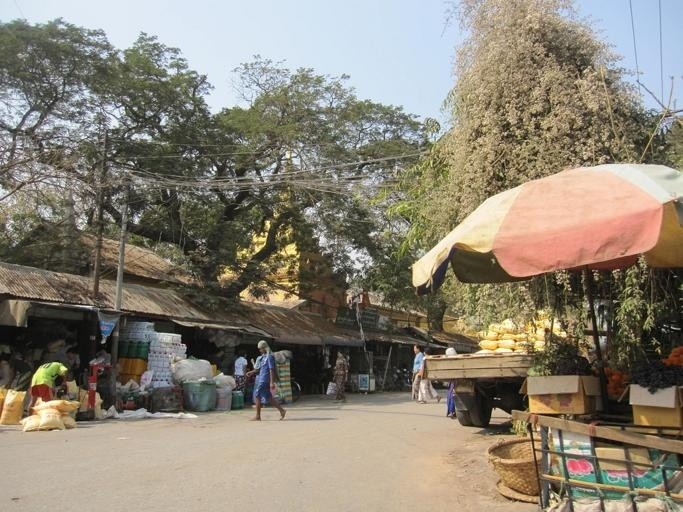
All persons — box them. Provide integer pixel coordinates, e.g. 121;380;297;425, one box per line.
234;349;248;392
332;351;349;402
246;340;286;422
417;347;441;404
66;345;81;370
27;359;69;416
411;344;424;400
445;347;457;419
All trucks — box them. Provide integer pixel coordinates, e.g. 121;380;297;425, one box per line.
424;349;545;429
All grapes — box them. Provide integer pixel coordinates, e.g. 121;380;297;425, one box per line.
636;360;683;394
557;358;586;375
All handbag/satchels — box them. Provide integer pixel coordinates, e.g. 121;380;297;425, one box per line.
326;381;337;396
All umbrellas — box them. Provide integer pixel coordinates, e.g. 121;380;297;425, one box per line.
408;162;683;416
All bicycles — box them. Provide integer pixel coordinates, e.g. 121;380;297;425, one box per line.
290;376;302;403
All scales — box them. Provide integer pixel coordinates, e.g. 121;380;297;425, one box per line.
75;357;105;421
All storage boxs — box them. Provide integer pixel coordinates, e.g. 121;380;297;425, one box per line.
549;425;681;501
615;383;682;431
513;371;604;416
118;319;186;389
